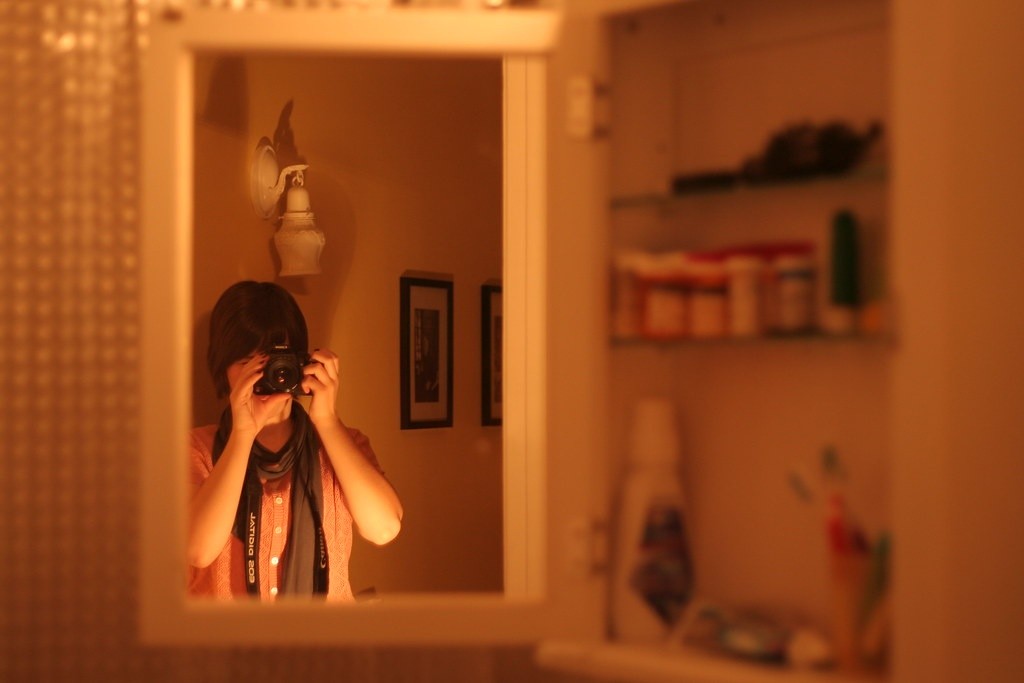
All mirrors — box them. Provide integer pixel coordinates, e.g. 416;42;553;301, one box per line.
139;12;550;651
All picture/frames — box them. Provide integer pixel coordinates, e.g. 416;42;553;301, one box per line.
480;281;503;426
399;275;456;430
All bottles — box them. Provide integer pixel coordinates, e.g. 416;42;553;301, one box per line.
609;391;695;649
609;241;815;336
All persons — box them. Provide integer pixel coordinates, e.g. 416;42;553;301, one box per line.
189;281;404;603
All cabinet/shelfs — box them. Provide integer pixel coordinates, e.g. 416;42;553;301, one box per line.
600;166;887;350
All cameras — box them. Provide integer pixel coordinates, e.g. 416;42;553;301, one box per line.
253;345;324;397
